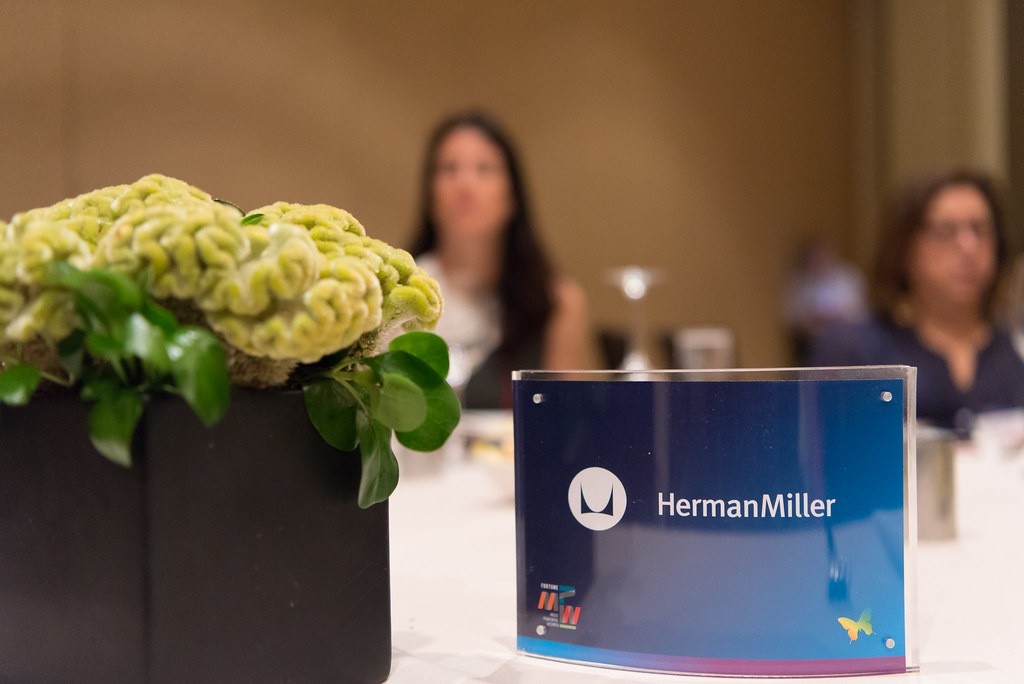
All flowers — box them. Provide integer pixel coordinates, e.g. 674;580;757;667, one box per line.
0;171;462;508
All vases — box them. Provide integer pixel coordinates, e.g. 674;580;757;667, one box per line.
0;388;392;684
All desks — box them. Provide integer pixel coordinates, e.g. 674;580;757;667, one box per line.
388;440;1024;684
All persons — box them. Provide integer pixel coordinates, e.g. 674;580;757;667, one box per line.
801;168;1024;429
377;107;605;410
